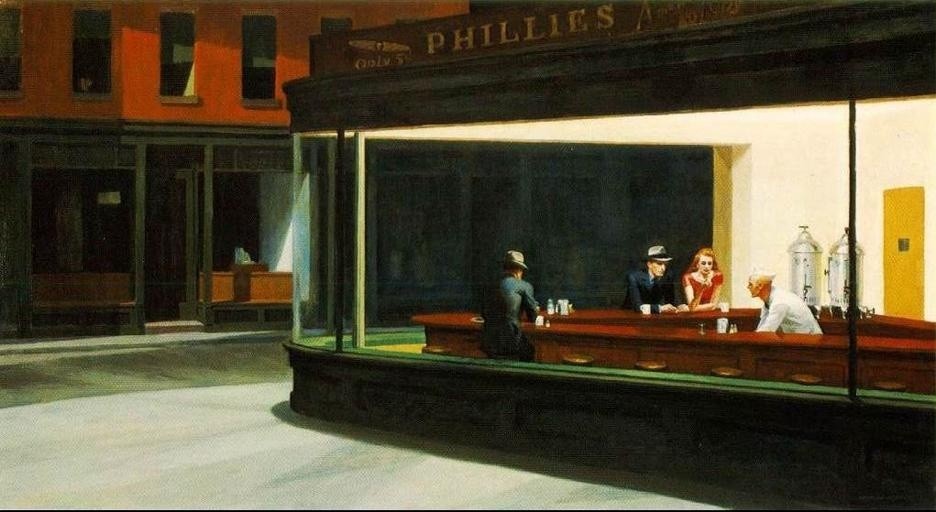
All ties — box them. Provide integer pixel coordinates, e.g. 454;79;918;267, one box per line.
653;278;660;286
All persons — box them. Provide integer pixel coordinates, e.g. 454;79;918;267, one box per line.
745;266;824;335
620;245;690;314
476;250;541;365
679;247;732;314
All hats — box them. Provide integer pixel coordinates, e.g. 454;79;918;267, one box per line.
505;250;528;270
647;245;672;261
749;266;776;280
